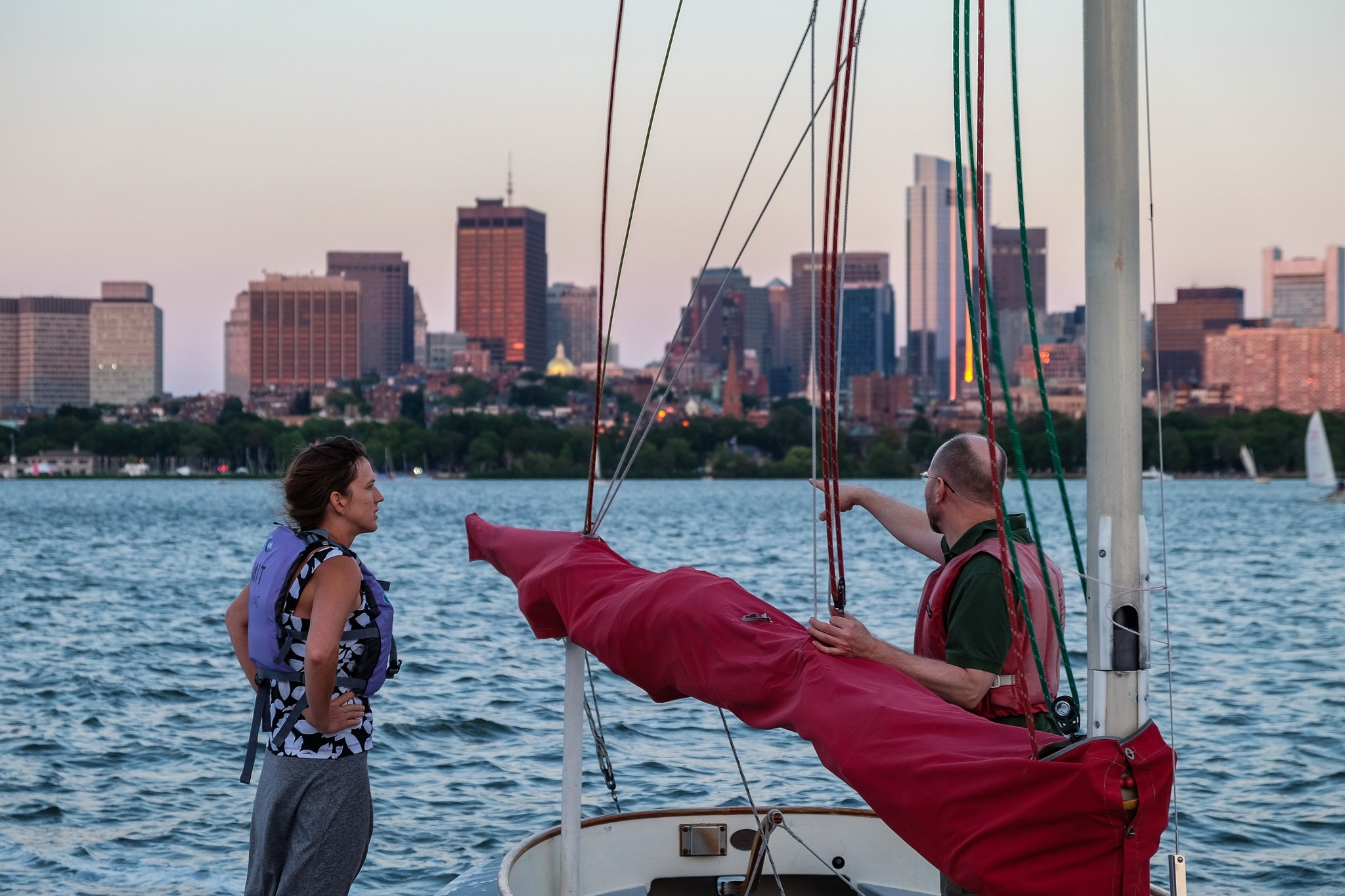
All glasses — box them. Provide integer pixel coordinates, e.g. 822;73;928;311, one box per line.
920;471;955;493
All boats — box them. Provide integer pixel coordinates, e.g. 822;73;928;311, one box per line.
1143;466;1174;481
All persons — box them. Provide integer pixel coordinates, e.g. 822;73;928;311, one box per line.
806;432;1063;896
224;435;404;896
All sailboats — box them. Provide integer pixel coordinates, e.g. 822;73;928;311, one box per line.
431;0;1183;896
1238;444;1269;484
1305;410;1344;505
